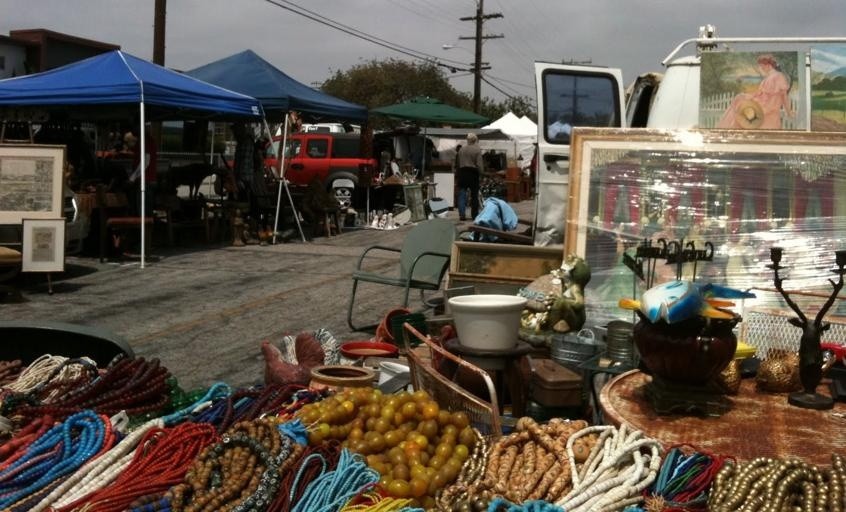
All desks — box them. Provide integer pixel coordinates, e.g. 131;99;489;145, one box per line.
442;335;533;418
69;193;129;236
600;355;846;467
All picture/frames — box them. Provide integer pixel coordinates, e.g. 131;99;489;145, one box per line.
20;216;68;273
0;143;66;226
561;125;846;372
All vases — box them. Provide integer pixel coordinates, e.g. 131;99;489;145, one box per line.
309;308;412;395
448;293;528;349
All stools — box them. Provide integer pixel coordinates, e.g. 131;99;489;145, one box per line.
309;206;347;238
0;246;23;302
105;217;155;259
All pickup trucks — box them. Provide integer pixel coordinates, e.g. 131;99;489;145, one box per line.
215;130;383;199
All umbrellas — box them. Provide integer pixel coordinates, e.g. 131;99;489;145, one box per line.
367;94;490;180
480;106;539;169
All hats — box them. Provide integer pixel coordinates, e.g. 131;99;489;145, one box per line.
734;98;764;130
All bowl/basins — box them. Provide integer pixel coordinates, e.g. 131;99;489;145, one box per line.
339;340;400;361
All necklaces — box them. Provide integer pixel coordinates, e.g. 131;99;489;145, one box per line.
1;347;846;512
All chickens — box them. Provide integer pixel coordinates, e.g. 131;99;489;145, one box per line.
260;331;325;388
279;327;341;366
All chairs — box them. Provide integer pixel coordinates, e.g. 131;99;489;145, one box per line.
0;319;135;367
402;321;528;440
345;217;458;332
155;192;211;244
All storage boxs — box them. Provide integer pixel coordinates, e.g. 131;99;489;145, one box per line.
519;359;583;407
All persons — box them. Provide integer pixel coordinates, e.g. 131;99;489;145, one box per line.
530;146;537;188
230;122;254;199
717;54;800;130
126;114;158;215
453;145;462;185
459;132;485;221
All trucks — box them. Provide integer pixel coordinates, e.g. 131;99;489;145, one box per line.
532;31;845;252
